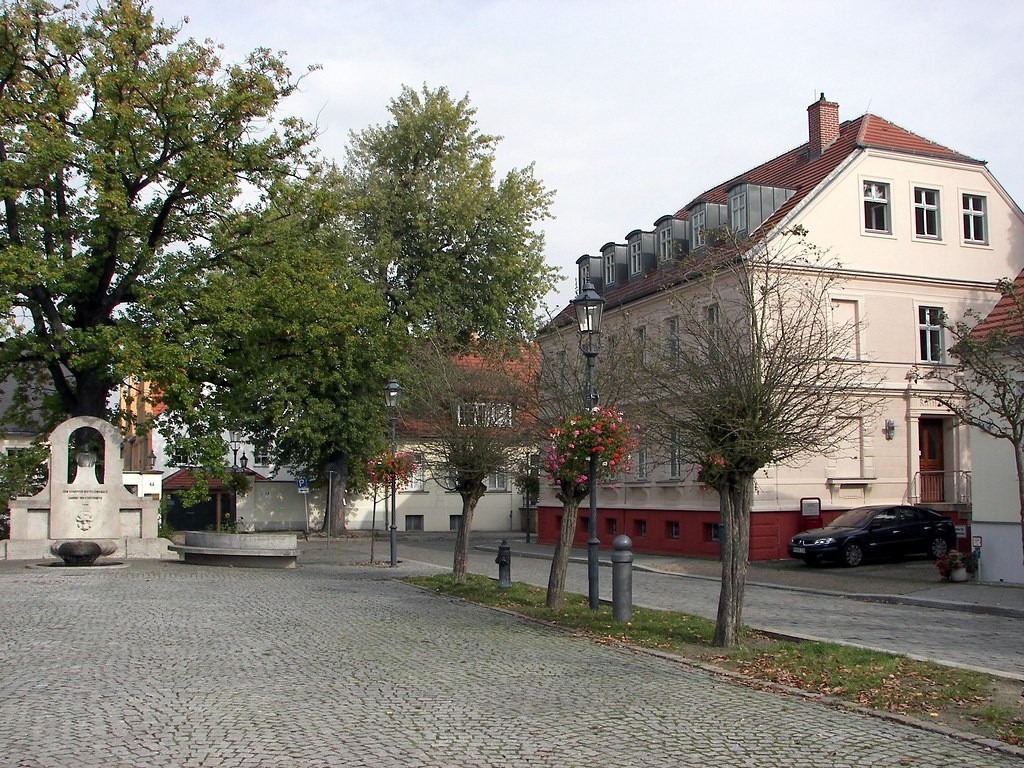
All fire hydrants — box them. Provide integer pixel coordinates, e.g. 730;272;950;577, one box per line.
495;540;511;587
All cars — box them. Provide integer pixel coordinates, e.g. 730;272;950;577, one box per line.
789;503;957;567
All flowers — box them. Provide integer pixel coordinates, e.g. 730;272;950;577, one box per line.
695;449;728;494
935;550;978;576
546;405;641;504
367;451;418;492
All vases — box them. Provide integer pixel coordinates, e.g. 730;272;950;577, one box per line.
950;568;968;581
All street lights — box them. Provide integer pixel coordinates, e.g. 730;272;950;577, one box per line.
569;278;607;612
383;377;403;570
522;446;533;542
230;438;242;533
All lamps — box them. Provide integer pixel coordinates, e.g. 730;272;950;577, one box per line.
886;419;894;440
972;536;982;548
148;450;157;469
